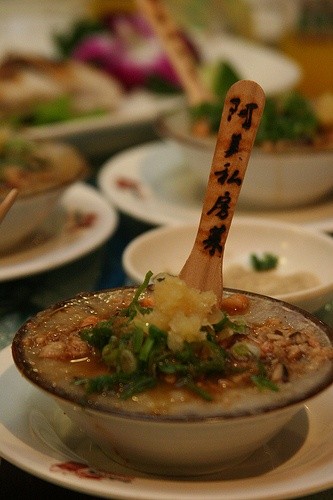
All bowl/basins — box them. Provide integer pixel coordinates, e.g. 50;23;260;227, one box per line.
1;139;89;252
10;284;333;476
122;217;333;315
158;100;333;208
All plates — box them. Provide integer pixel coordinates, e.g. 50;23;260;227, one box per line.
1;344;333;500
96;139;333;233
1;180;119;282
0;13;304;159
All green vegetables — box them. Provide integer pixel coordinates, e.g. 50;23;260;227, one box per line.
187;64;325;141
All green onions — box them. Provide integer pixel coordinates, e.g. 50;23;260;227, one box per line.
71;270;281;402
